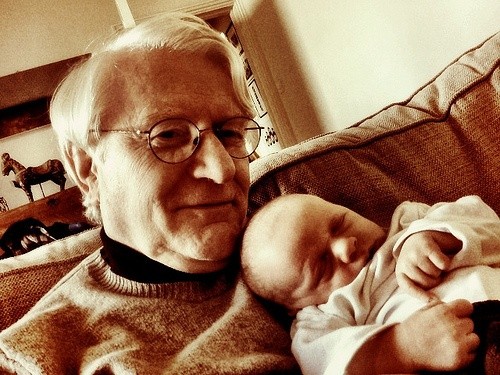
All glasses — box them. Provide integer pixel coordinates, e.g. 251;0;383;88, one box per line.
88;116;264;166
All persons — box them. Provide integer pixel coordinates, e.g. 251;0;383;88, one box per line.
240;193;500;375
0;9;308;375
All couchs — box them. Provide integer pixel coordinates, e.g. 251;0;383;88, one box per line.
1;31;500;374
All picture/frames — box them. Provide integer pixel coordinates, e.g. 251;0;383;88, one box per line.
223;19;245;59
235;51;253;84
245;80;268;119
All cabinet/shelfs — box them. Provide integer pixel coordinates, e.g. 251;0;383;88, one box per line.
1;91;102;261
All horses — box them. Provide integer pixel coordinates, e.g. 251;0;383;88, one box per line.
0;153;66;203
